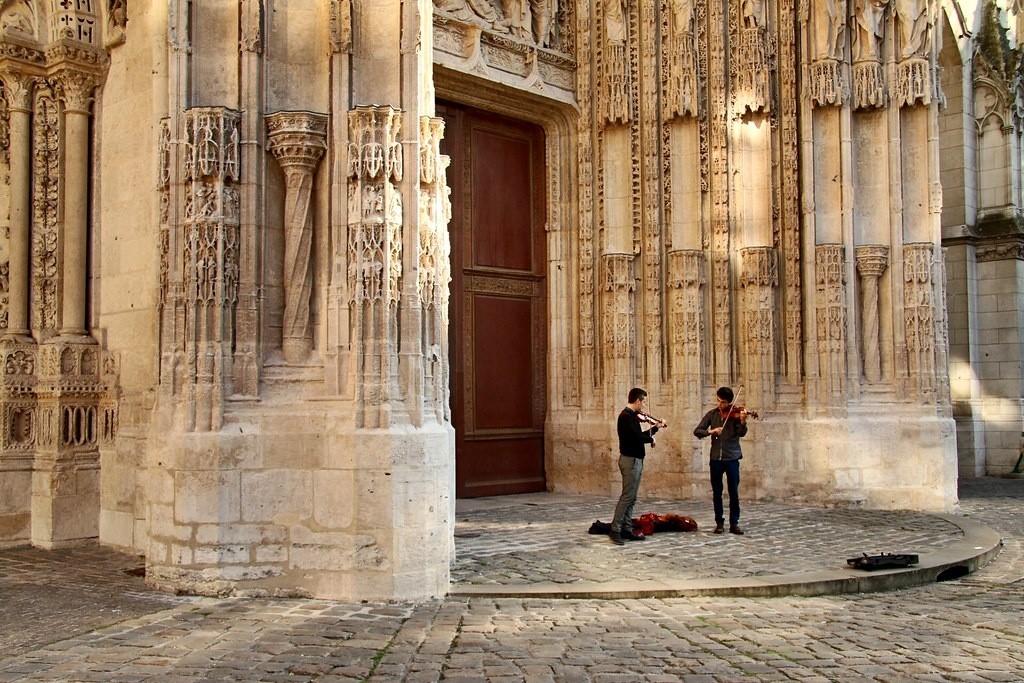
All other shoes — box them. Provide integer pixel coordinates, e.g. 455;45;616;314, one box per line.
714;523;724;533
621;531;640;540
730;523;744;535
609;530;624;545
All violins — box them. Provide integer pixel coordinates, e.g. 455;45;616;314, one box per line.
720;404;759;421
636;410;667;428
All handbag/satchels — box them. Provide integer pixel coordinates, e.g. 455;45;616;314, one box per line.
588;520;610;534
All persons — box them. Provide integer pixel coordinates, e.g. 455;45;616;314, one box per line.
693;386;748;535
609;387;666;546
